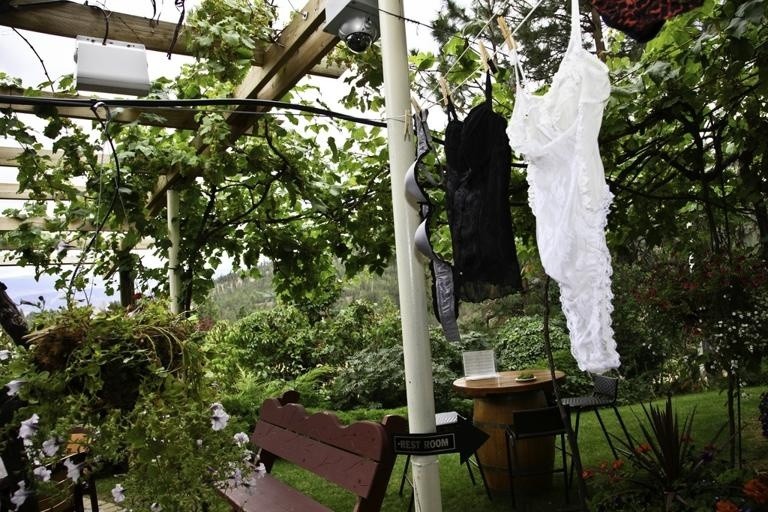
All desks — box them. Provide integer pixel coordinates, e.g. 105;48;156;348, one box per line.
450;366;574;508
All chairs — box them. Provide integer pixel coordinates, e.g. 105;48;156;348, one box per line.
555;371;643;491
0;442;107;512
395;409;481;503
501;404;581;511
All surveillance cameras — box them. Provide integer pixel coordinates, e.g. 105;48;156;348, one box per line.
337;16;378;52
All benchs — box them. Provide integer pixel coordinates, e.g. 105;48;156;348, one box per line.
202;395;406;512
